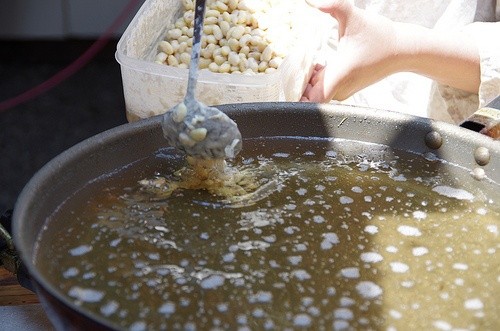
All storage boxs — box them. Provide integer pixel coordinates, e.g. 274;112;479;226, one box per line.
113;0;329;124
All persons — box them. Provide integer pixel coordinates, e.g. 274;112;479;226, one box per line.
299;0;500;126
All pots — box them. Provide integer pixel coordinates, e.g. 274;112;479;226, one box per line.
0;104;500;331
